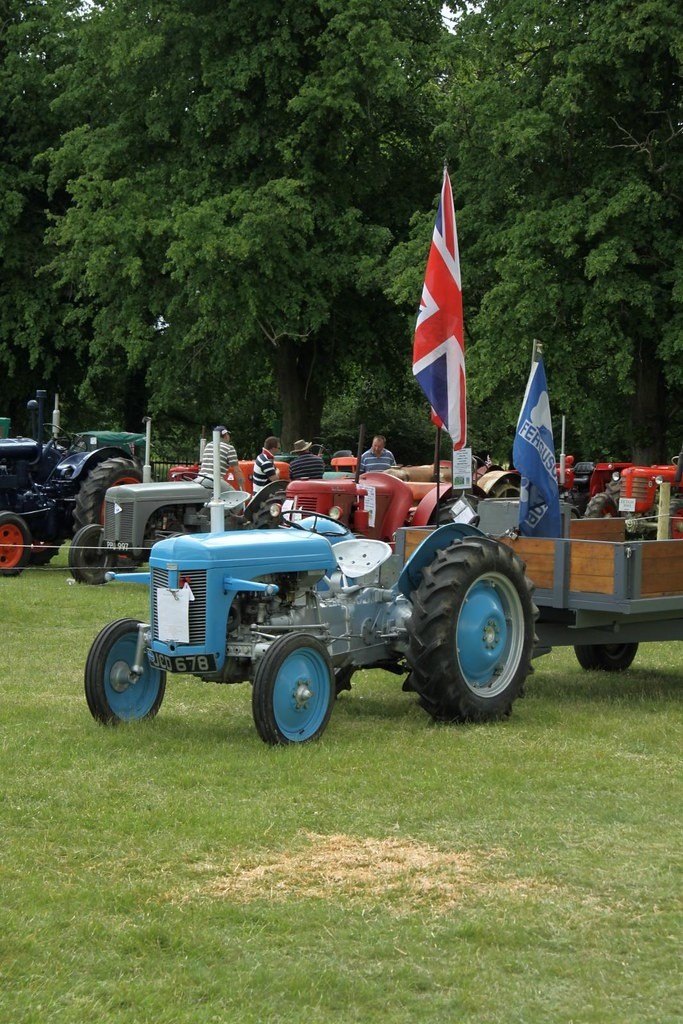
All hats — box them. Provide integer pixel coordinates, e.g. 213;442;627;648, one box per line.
290;440;312;452
214;426;231;436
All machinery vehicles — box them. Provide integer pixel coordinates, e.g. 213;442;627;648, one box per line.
68;417;287;585
581;446;683;539
555;415;634;519
83;431;683;748
0;390;158;577
269;423;487;554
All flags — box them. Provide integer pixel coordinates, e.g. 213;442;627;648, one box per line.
514;358;561;538
412;173;468;452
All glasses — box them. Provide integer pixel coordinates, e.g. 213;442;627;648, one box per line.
276;447;281;450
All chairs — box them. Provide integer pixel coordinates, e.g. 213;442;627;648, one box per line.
332;539;393;594
216;491;252;514
57;452;91;480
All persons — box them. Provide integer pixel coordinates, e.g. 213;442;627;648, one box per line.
253;436;282;496
198;426;246;514
288;439;324;480
359;435;397;473
